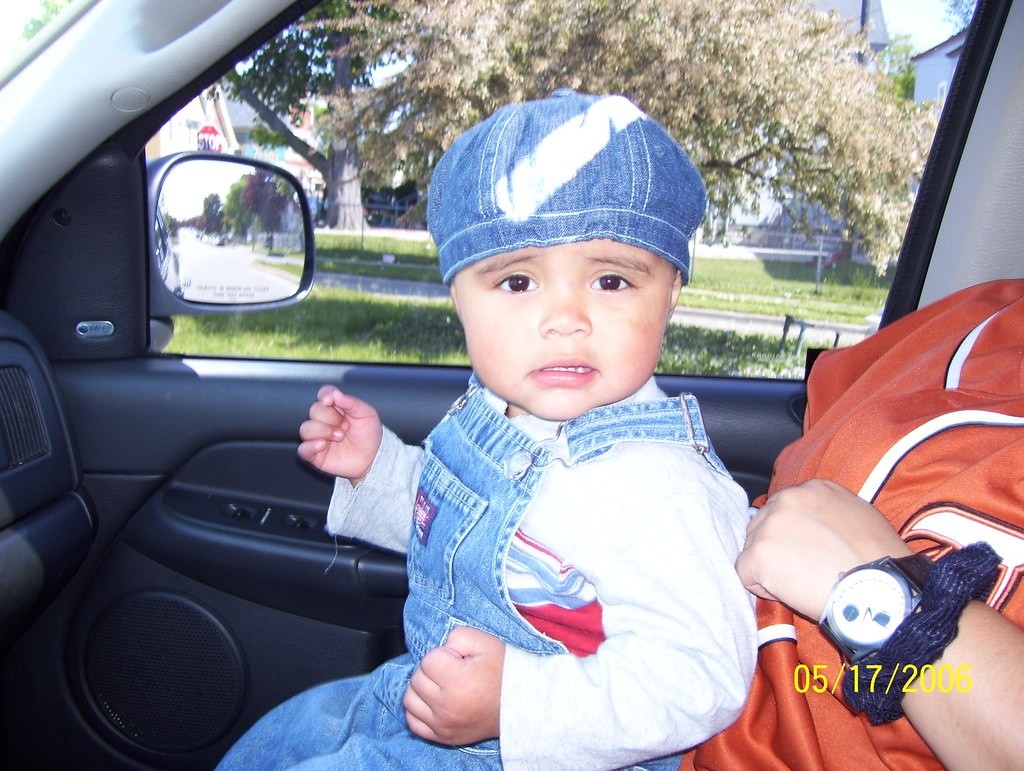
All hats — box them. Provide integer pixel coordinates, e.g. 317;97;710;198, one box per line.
427;89;707;288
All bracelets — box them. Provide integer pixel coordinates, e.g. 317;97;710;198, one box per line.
843;542;997;724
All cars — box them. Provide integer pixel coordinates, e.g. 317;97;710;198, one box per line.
155;207;184;299
214;235;224;246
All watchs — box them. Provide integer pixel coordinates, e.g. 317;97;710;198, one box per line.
818;554;931;663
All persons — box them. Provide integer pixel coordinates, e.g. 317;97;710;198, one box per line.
678;278;1024;771
217;90;759;771
